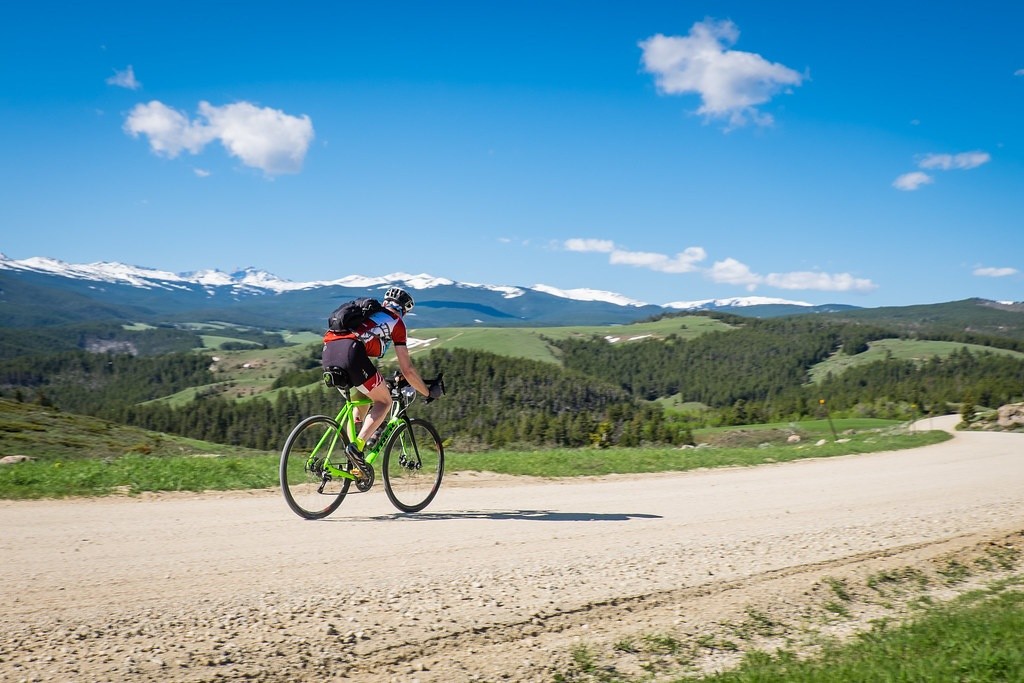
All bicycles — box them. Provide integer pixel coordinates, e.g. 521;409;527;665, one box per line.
278;367;447;520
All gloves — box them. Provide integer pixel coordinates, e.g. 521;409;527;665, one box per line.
428;385;442;399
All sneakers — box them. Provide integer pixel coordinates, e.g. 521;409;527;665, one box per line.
346;444;368;477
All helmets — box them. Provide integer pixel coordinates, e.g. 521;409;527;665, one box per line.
384;287;414;312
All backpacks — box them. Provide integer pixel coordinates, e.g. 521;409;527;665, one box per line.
327;297;397;349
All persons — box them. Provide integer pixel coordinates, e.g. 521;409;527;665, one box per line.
321;286;441;479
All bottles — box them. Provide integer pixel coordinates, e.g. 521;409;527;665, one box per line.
367;421;388;449
